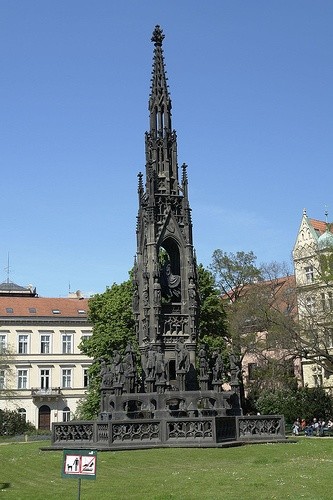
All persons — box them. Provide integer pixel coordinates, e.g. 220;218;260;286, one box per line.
307;418;325;437
99;337;167;386
292;418;300;435
133;255;197;307
326;419;333;431
197;344;241;383
300;418;306;431
175;336;189;370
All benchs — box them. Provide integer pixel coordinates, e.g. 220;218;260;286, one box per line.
292;425;333;436
